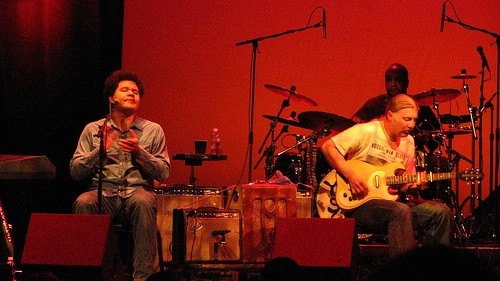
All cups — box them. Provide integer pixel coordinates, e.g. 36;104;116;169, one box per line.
194;140;207;154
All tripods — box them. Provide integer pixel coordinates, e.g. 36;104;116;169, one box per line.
446;64;499;245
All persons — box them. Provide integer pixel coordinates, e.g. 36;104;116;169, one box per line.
70;70;171;281
351;63;442;152
320;94;452;259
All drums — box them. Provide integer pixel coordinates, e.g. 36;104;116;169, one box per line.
272;152;303;180
419;152;452;197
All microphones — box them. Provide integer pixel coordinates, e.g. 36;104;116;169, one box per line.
108;99;119;116
210;230;231;235
440;5;446;31
323;10;326;38
281;110;298;133
477;47;492;71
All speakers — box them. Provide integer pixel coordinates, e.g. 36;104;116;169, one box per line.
155;188;226;263
18;212;120;281
271;218;358;279
183;211;241;262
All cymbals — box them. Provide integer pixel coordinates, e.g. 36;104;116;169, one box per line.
450;75;477;79
430;126;476;138
298;111;357;131
263;84;318;107
261;113;313;130
412;87;462;104
441;114;477;123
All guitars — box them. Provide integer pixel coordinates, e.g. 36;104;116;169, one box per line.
334;160;485;211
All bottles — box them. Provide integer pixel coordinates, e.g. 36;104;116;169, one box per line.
211;128;221;155
